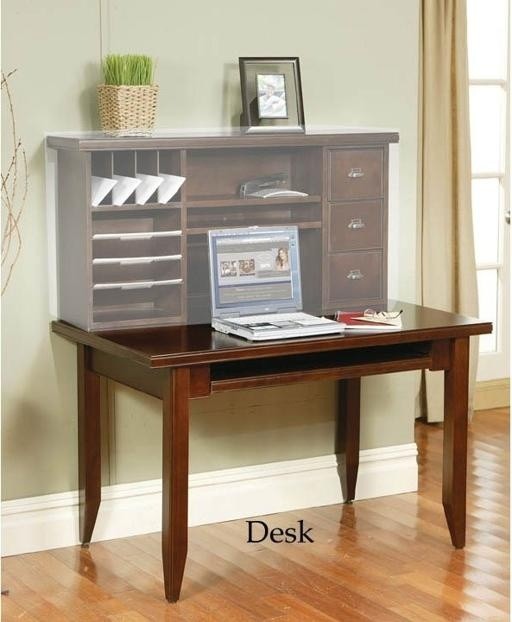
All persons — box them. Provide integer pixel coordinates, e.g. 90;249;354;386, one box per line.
274;247;290;271
259;85;286;112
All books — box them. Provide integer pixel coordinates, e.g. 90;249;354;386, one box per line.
335;310;402;331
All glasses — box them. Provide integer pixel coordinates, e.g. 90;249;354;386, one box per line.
363;307;404;320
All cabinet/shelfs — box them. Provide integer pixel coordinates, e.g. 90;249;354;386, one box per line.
46;129;403;335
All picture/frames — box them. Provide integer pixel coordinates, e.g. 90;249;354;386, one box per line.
237;55;306;135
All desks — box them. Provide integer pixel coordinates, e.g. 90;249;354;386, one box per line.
49;297;495;605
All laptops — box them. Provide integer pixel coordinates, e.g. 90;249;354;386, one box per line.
207;226;347;342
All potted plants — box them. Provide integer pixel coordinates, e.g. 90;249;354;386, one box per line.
95;51;160;138
